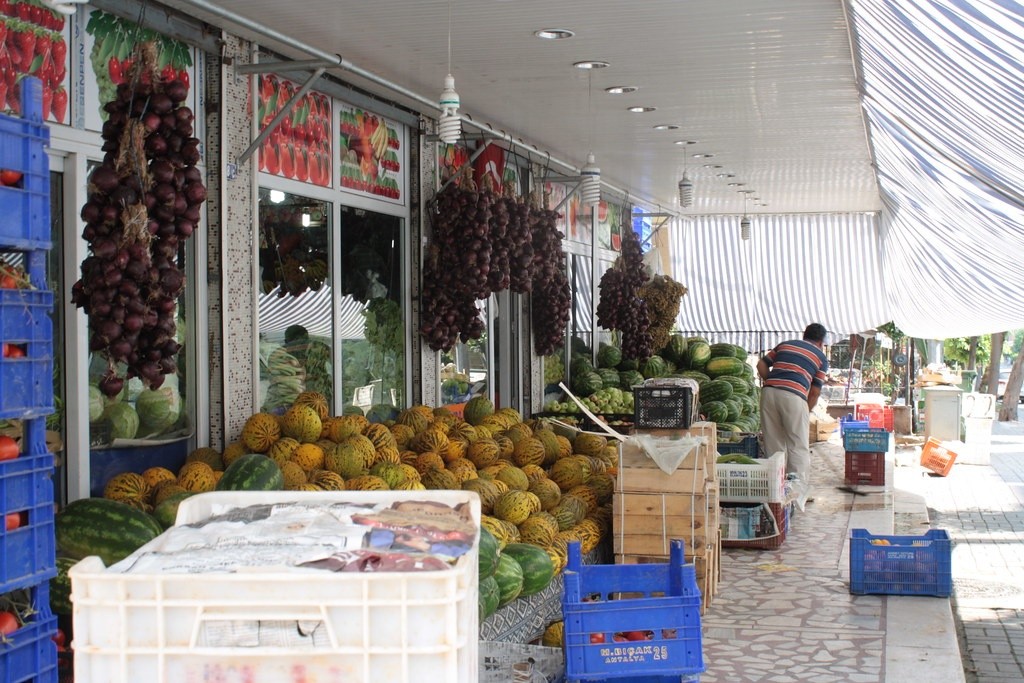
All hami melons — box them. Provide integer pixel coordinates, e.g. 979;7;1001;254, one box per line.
102;392;621;647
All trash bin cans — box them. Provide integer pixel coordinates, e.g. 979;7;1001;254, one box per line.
963;391;995;465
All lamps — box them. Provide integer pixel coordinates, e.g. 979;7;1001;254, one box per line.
740;194;749;242
678;145;693;209
438;0;461;144
581;70;600;206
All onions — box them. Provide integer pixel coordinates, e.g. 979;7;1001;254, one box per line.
71;80;207;399
420;176;571;357
597;231;655;361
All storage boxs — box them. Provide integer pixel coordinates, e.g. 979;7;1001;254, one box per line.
67;491;481;683
0;416;58;594
0;76;54;250
0;251;55;419
0;580;58;683
613;421;722;616
716;452;785;502
845;427;888;452
849;528;951;597
920;436;957;477
560;539;706;680
840;404;894;438
633;385;700;428
719;502;786;551
844;451;885;486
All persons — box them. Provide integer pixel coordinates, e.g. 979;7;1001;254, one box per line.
757;323;827;502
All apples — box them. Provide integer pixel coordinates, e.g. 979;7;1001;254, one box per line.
545;386;635;427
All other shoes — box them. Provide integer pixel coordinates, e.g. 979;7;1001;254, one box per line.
805;495;814;502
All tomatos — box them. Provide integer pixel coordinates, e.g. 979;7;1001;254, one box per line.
0;167;68;656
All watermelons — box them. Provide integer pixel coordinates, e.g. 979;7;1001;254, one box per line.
42;453;554;630
570;334;763;443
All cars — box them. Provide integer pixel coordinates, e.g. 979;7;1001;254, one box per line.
997;369;1024;404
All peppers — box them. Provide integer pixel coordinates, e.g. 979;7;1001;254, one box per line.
338;107;400;199
0;0;189;124
245;73;332;187
